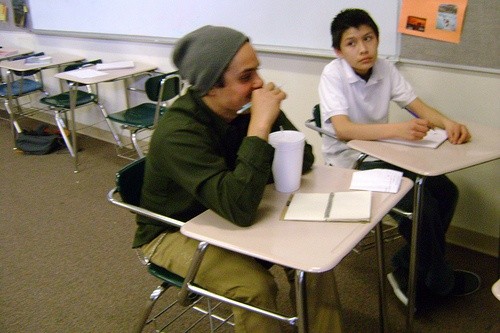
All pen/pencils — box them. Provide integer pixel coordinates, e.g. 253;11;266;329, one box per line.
404;107;435;131
236;83;282;114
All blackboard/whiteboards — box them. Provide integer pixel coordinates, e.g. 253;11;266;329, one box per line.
24;1;401;66
399;1;499;75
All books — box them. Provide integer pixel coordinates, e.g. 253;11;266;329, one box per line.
381;128;449;148
24;57;51;64
280;191;371;222
97;61;134;69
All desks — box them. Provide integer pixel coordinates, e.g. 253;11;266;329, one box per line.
0;45;500;333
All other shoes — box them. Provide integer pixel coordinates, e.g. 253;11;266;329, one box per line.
387;268;481;313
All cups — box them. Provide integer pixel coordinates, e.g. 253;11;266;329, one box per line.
268;131;306;193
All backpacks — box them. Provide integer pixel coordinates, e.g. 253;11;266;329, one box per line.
16;125;70;155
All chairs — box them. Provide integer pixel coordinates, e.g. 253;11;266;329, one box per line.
107;70;184;159
107;157;235;333
39;59;122;159
0;51;52;133
304;104;403;252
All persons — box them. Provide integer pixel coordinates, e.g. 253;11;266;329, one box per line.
132;25;346;333
319;9;482;312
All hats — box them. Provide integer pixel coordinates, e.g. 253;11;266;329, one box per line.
172;25;249;98
